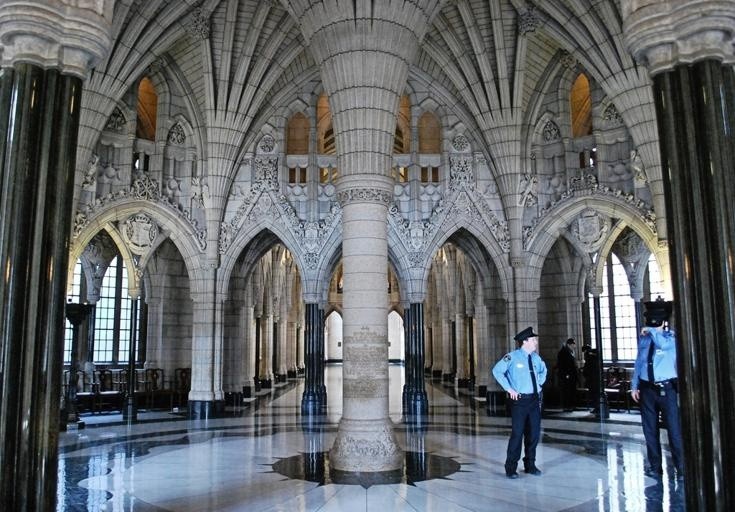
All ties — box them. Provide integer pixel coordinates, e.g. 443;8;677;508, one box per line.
646;340;656;385
527;354;539;395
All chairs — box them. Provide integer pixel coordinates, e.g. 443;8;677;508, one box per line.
119;369;148;412
577;367;589;409
604;367;626;411
625;380;631;414
65;370;94;415
146;368;172;412
174;368;191;410
93;369;122;414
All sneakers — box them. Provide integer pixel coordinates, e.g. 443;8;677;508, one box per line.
644;467;663;480
525;467;542;475
678;471;685;482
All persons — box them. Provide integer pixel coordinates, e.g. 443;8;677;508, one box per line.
627;310;685;481
581;344;601;415
556;338;579;411
491;326;546;480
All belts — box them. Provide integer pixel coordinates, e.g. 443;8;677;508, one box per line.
639;377;674;390
516;393;538;400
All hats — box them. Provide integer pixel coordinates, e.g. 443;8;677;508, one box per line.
513;326;541;344
642;310;668;329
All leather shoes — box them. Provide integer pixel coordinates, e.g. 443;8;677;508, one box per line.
506;468;520;479
